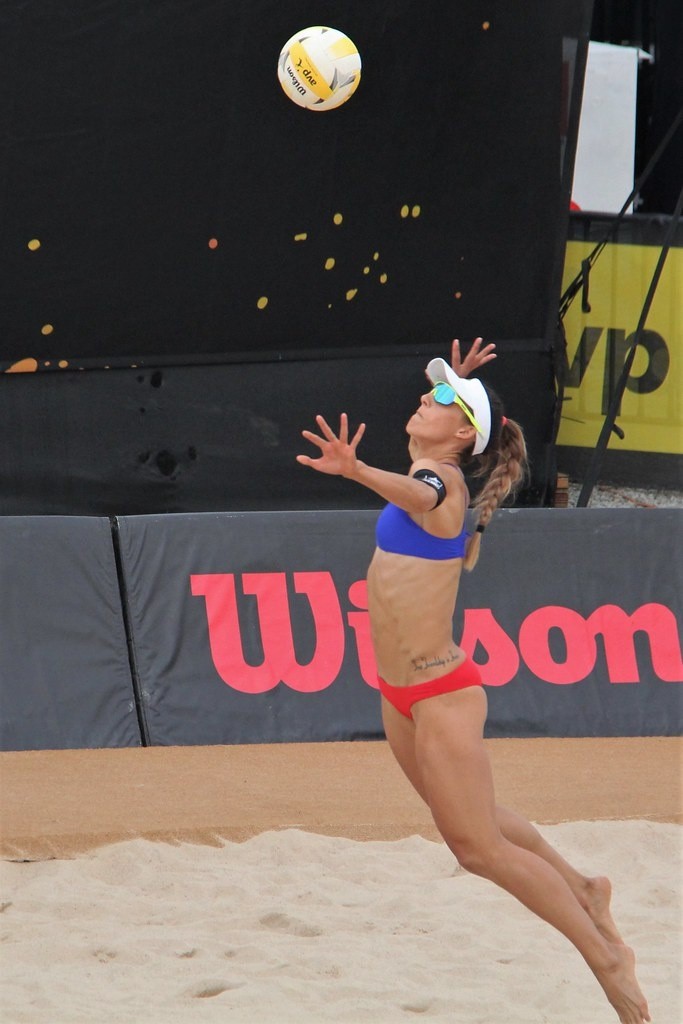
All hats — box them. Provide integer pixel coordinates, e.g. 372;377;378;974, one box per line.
426;358;492;456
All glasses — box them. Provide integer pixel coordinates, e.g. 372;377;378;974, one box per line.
430;382;486;438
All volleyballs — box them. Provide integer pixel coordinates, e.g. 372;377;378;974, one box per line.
277;26;362;111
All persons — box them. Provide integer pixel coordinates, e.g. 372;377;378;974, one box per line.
295;337;652;1024
